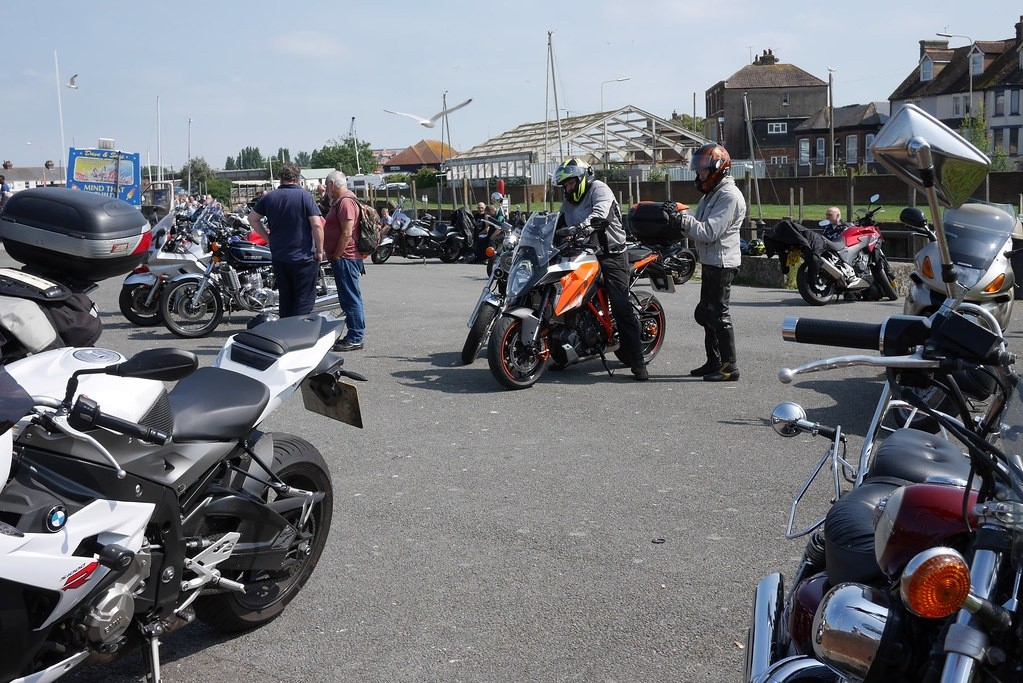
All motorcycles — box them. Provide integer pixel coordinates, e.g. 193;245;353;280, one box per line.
0;312;367;683
0;187;154;367
462;193;525;365
118;199;698;338
487;210;682;390
742;99;1023;682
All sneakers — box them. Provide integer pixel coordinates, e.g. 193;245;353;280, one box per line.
632;364;649;382
690;358;741;382
547;361;563;372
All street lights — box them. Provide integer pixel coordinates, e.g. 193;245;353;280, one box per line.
936;32;974;136
600;76;631;113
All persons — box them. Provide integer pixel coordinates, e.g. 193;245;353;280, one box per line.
547;158;649;380
475;203;490;263
0;175;10;205
380;208;392;234
317;185;330;217
248;165;325;318
322;172;365;351
823;208;851;238
662;145;746;380
485;205;505;241
251;190;267;202
176;194;213;215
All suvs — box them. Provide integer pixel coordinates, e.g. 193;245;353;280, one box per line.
378;183;409;191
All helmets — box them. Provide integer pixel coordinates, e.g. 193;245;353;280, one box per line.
747;238;766;256
551;157;596;206
690;142;732;195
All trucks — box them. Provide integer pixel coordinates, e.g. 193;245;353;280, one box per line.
66;145;174;224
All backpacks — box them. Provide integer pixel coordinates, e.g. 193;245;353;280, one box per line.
334;194;382;255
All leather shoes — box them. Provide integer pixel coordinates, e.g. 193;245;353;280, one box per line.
333;336;364;353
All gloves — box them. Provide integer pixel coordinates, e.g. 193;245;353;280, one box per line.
656;200;683;232
